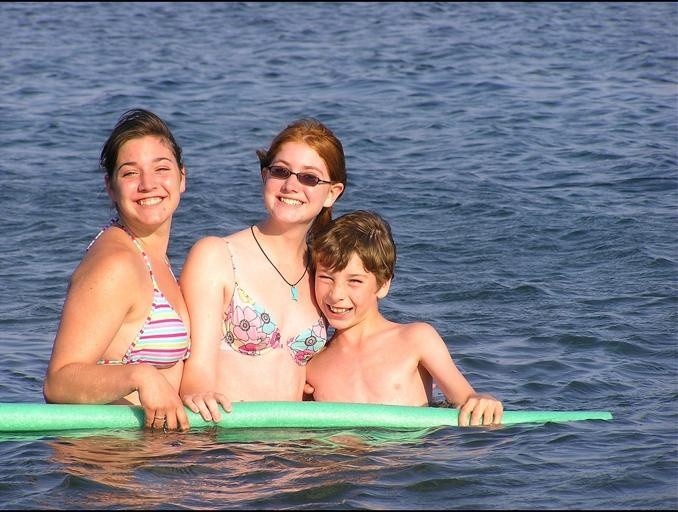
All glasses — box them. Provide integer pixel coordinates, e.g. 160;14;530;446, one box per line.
267;166;332;186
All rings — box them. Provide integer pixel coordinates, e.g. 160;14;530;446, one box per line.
154;416;167;420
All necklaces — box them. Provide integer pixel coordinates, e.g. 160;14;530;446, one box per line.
251;223;309;301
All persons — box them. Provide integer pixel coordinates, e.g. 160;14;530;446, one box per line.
44;108;191;432
304;211;502;432
179;116;349;423
